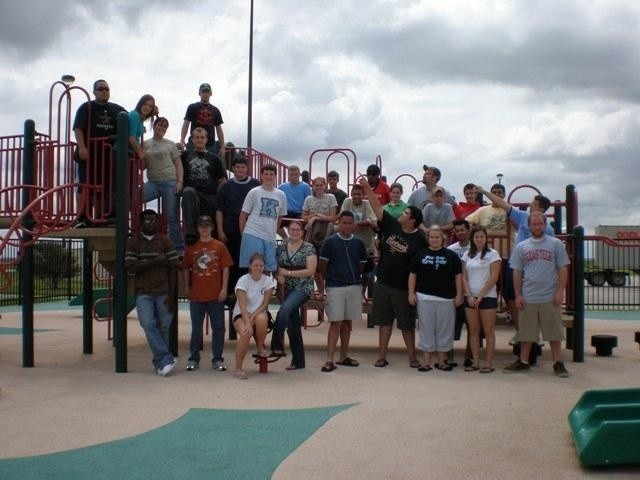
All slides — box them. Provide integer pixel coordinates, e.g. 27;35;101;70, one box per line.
563;388;639;470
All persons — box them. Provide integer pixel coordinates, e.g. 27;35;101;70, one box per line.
73;77;570;377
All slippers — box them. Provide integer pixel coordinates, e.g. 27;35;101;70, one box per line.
320;361;337;372
479;366;495;373
231;370;249;381
465;366;480;372
437;363;452;371
408;360;422;369
336;359;359;367
375;359;388;367
259;350;269;357
418;364;432;370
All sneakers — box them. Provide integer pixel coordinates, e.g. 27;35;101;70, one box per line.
464;359;472;367
285;364;296;371
272;349;285;356
504;358;530;372
553;361;569;378
213;359;227;372
157;358;177;378
434;361;458;368
508;330;545;347
186;361;200;371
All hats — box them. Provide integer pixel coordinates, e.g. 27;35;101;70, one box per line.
421;164;441;181
196;214;213;225
199;83;212;92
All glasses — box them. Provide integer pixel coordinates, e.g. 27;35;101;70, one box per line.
96;86;110;91
144;219;158;223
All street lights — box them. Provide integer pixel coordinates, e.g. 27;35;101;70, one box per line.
61;74;77;300
496;173;504;185
